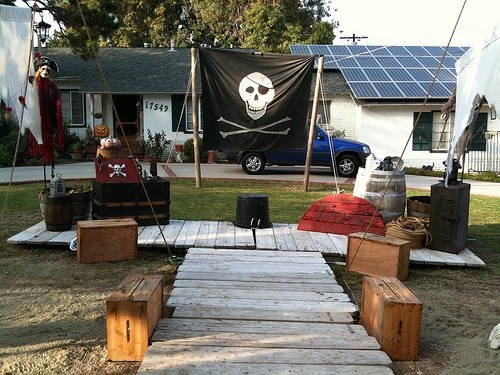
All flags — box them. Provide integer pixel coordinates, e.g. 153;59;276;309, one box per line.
198;47;316;149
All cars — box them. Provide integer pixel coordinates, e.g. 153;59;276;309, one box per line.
223;119;371;178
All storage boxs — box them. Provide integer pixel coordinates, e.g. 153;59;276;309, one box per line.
347;231;410;281
107;273;164;362
76;218;137;263
360;277;423;361
91;180;171;226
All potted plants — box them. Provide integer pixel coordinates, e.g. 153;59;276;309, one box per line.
72;143;87;160
81;124;99;153
407;196;430;218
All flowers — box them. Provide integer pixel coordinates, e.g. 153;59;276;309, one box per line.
67;184;93;193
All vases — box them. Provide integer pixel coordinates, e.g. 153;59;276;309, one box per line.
176;144;184;153
39;188;92;223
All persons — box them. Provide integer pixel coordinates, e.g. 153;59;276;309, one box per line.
26;56;63;165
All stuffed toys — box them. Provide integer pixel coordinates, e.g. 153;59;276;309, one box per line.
100;137;121;149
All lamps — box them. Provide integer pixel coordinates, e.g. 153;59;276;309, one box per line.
491;106;496;120
